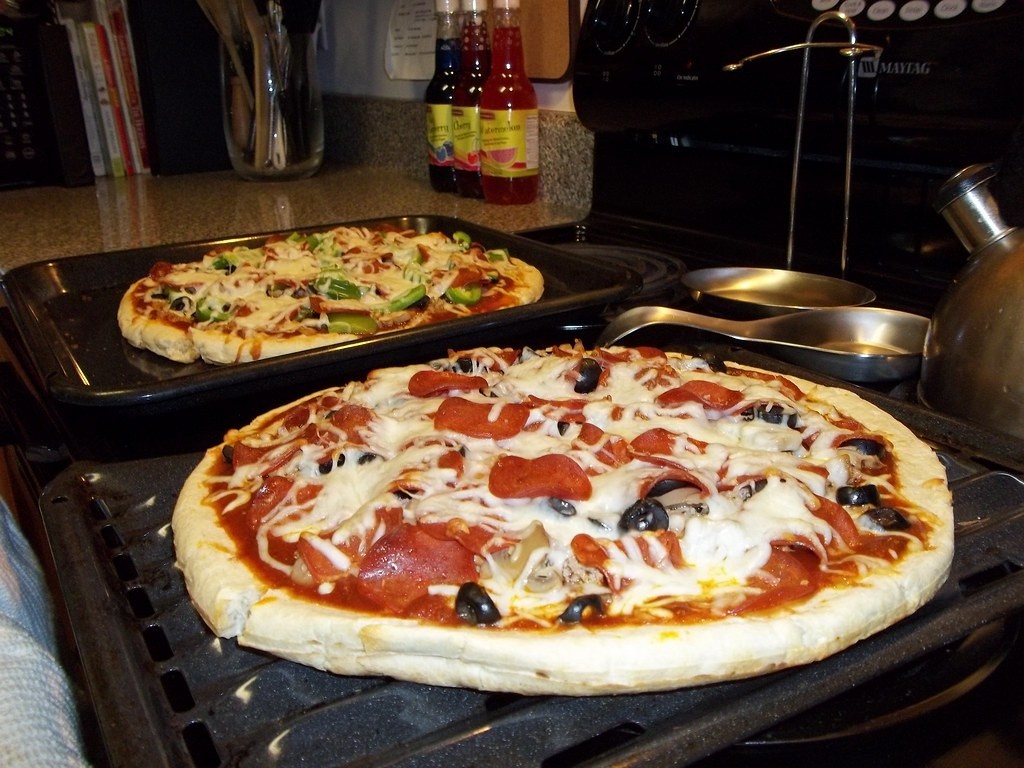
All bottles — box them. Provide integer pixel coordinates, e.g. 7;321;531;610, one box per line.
424;0;461;193
452;0;490;197
480;0;540;205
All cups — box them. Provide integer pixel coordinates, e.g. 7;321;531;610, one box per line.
214;18;325;177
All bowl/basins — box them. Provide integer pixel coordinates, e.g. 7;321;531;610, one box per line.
682;267;874;324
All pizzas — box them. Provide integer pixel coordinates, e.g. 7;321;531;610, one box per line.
171;338;955;697
116;225;545;367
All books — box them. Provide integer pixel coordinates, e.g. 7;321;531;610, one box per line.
0;0;152;187
